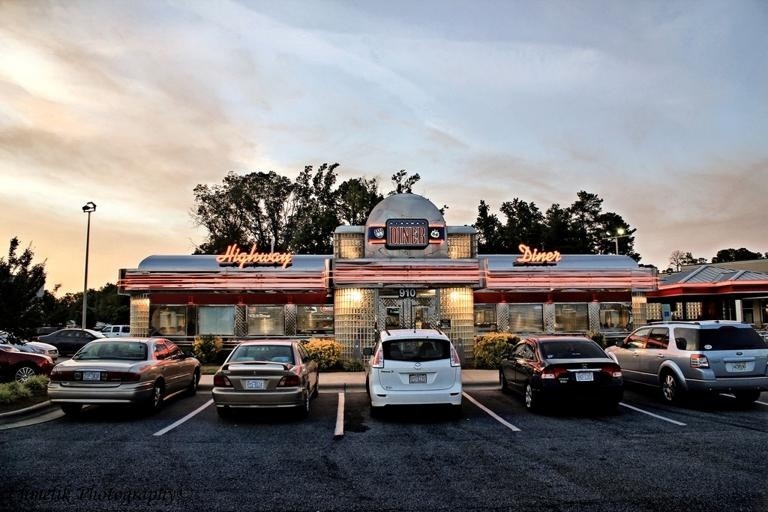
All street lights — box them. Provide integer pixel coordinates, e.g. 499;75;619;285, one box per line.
82;201;96;327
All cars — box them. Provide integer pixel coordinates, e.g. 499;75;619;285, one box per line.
363;329;462;411
38;325;130;354
211;340;319;413
47;338;200;414
499;320;768;409
0;330;58;386
